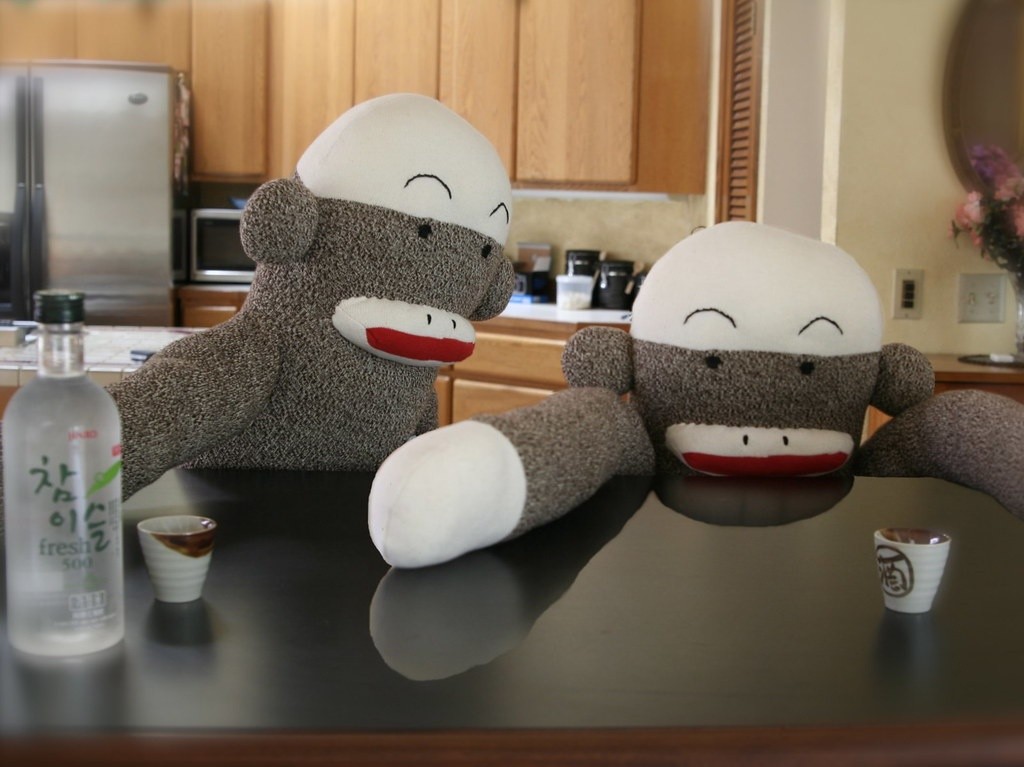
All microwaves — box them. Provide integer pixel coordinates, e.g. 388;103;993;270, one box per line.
190;209;257;284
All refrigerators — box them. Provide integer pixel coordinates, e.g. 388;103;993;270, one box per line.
1;60;189;329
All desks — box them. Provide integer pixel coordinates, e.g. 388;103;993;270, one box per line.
0;320;212;418
0;467;1024;767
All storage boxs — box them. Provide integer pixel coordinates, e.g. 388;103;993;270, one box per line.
508;242;553;305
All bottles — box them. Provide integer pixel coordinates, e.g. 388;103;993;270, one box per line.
0;289;124;657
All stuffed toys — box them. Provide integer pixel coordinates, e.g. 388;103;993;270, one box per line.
367;220;1024;568
95;90;516;504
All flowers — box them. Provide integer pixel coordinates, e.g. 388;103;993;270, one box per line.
943;141;1024;292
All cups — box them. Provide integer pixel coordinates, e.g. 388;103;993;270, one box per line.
556;276;595;308
565;249;601;276
138;516;216;602
595;261;635;310
873;527;951;613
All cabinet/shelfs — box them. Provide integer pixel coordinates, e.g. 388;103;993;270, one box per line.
0;0;715;196
174;281;635;434
854;338;1023;450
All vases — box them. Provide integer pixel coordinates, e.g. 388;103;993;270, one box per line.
1003;270;1024;361
